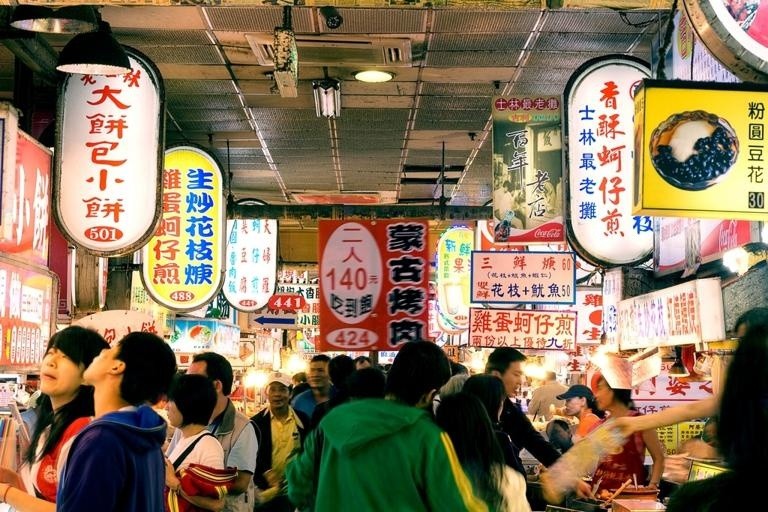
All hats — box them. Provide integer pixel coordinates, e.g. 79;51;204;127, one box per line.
268;370;292;388
556;385;594;402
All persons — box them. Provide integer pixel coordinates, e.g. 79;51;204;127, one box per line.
0;325;110;512
601;306;767;512
52;330;174;509
157;376;226;510
249;340;665;511
187;351;261;510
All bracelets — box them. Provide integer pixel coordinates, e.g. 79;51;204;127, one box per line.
4;485;12;505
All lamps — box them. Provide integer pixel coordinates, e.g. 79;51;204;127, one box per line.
56;19;131;76
10;4;100;35
312;78;341;120
